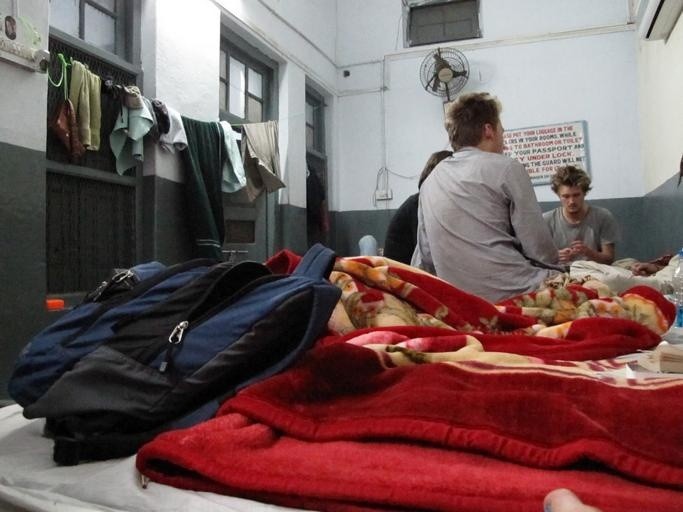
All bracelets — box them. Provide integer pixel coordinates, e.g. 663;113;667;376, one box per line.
655;264;660;272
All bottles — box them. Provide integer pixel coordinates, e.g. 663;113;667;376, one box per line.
672;250;683;328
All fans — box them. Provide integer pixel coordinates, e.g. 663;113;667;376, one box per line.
419;47;470;113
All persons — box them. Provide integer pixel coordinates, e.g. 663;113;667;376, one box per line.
383;150;453;265
632;156;683;275
410;91;560;304
541;164;616;272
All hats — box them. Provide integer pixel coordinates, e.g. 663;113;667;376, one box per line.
123;85;145;109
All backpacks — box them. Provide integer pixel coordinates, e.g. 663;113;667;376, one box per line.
9;242;342;467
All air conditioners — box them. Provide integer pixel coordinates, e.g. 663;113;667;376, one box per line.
634;0;683;41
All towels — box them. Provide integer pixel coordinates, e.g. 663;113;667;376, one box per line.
217;119;249;194
159;104;188;156
71;59;102;151
110;102;157;178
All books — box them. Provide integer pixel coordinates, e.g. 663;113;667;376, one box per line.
625;346;683;378
